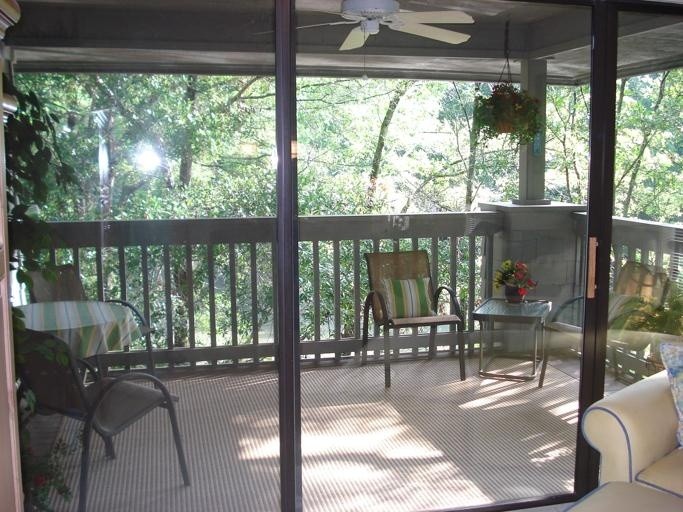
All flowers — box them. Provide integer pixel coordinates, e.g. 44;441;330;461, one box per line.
15;385;84;512
470;83;540;151
492;258;538;297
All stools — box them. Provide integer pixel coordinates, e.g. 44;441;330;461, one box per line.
562;482;683;512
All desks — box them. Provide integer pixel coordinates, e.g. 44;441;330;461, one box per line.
472;297;552;381
13;300;140;383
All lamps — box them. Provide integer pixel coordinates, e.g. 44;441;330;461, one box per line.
358;17;379;82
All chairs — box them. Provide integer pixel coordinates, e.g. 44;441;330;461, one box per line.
539;263;669;389
362;250;465;388
13;327;191;512
581;367;683;502
17;263;156;378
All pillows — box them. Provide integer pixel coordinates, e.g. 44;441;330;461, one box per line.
607;291;636;326
379;277;436;318
660;343;683;451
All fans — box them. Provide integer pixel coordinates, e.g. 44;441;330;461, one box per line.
250;0;474;51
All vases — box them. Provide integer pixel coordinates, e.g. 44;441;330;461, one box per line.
492;107;518;134
507;282;524;304
